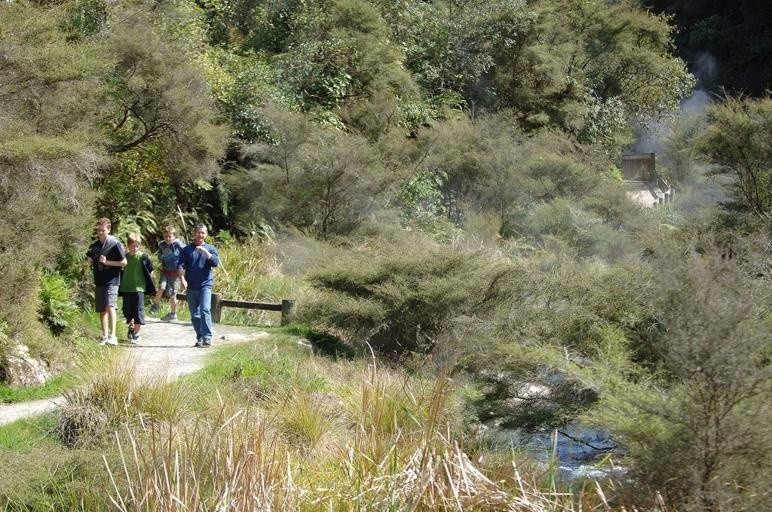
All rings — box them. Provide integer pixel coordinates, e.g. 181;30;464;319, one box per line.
184;284;185;285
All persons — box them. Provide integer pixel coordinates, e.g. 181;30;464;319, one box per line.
176;222;221;347
83;218;128;346
149;225;187;322
118;233;157;344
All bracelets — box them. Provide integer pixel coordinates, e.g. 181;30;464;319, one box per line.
201;249;207;255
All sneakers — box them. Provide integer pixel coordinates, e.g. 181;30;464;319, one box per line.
131;332;142;344
128;325;135;340
106;335;118;346
98;335;109;347
203;340;212;347
195;340;203;348
160;312;177;323
148;303;160;315
